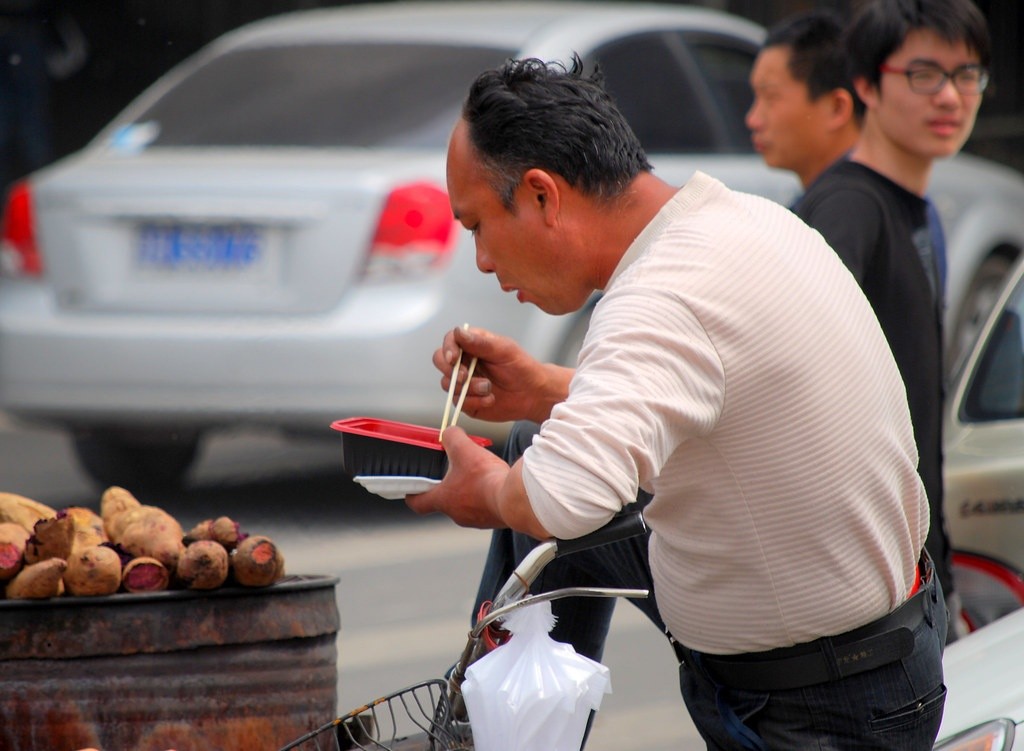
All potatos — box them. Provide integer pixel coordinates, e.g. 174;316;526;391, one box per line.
0;486;285;601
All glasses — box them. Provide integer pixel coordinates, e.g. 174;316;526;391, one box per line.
880;61;990;94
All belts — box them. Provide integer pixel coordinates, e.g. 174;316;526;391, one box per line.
666;580;935;692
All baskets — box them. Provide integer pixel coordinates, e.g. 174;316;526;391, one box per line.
276;677;470;751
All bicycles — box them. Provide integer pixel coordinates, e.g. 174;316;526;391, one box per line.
280;511;691;751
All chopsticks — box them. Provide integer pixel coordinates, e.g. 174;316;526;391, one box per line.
436;322;483;446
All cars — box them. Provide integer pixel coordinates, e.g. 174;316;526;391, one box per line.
930;258;1024;629
0;0;1024;500
928;607;1024;751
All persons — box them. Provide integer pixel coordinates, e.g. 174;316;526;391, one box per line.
405;53;948;751
794;0;995;646
748;15;947;330
